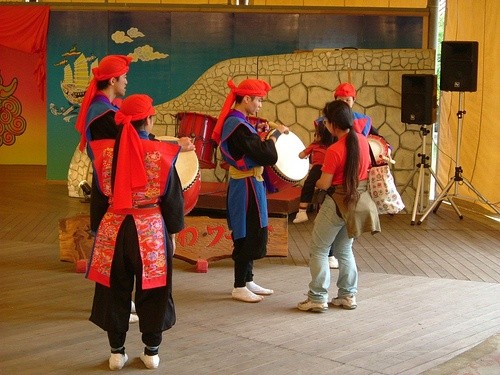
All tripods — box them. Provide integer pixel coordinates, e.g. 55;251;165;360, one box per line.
389;91;500;225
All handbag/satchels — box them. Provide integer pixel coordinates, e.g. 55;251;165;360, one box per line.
369;142;405;215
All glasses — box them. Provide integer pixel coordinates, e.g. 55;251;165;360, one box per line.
323;119;327;124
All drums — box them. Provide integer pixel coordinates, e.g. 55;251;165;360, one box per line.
257;128;309;190
153;135;201;216
367;135;388;166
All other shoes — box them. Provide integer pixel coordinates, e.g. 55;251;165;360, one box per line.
129;300;140;323
292;210;308;223
108;352;128;370
328;255;339;268
232;281;274;302
141;350;159;368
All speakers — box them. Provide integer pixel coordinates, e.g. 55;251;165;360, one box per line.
440;41;479;92
401;74;437;125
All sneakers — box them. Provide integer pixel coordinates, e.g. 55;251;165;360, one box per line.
332;293;357;309
297;296;329;312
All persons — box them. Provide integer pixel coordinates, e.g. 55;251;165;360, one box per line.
212;77;289;301
75;54;196;323
292;83;391;312
87;93;184;369
